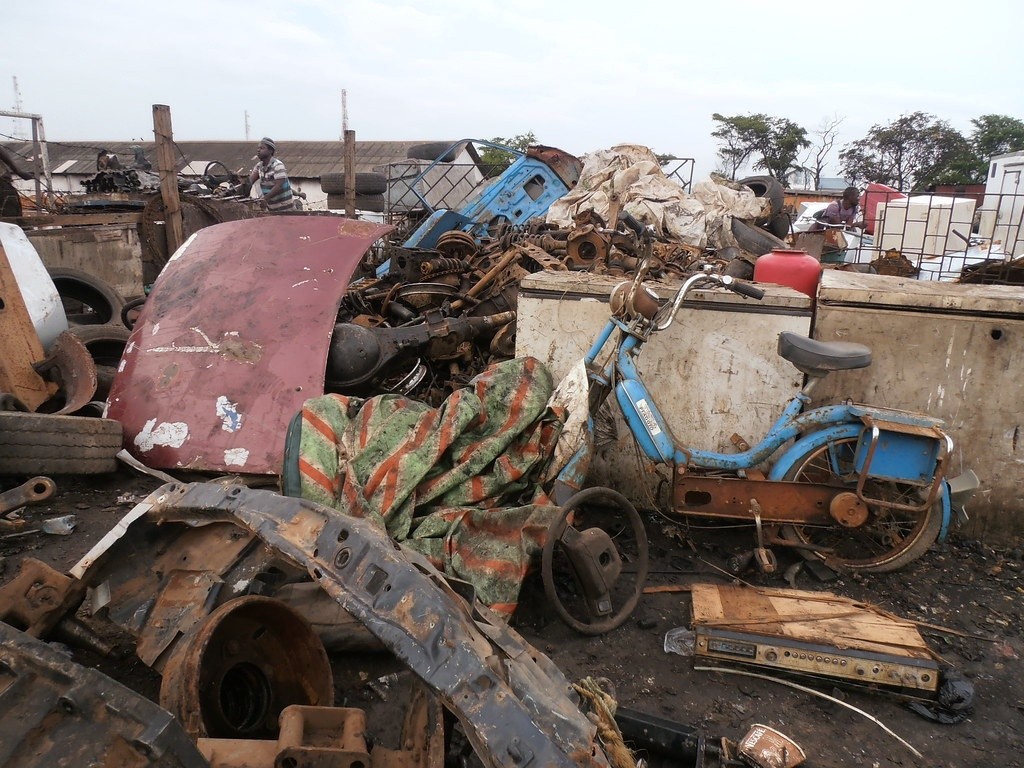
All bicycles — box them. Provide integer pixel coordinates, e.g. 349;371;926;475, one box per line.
548;209;957;575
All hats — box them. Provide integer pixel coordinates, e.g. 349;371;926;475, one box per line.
260;137;275;149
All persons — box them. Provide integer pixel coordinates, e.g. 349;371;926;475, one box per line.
0;160;22;217
213;137;306;212
809;187;868;232
